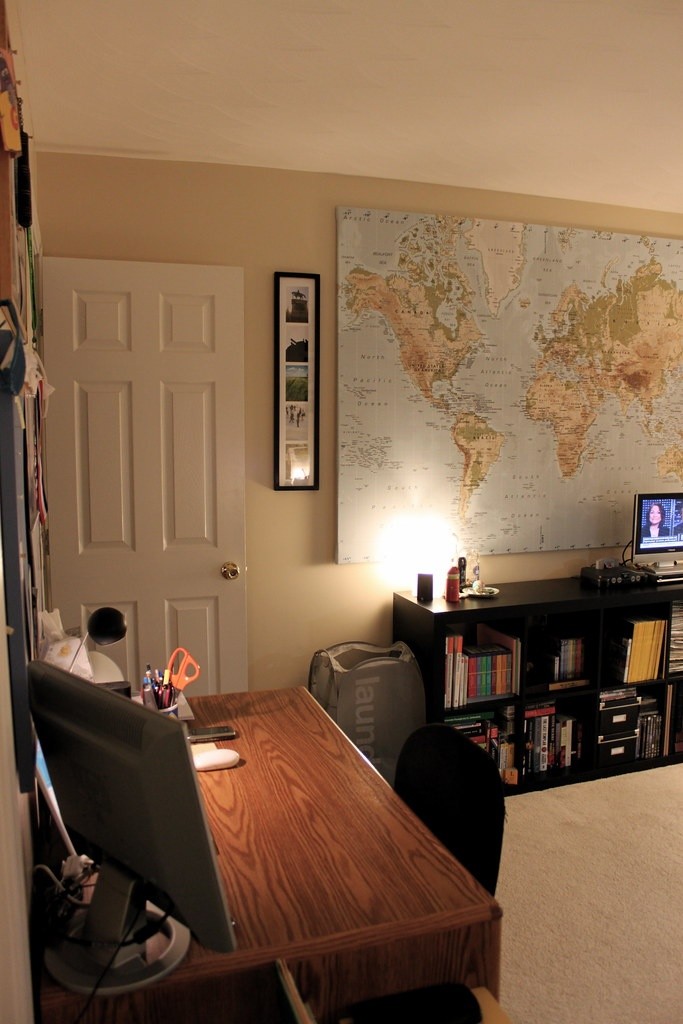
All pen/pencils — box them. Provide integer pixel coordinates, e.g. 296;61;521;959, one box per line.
139;663;177;708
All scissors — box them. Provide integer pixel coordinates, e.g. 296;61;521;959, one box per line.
167;648;201;707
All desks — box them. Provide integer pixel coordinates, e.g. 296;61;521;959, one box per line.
41;686;505;1024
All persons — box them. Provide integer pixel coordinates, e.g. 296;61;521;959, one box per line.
674;507;683;535
642;504;669;536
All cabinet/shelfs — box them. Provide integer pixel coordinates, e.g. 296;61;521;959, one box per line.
391;576;683;797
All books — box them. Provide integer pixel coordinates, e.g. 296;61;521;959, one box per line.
609;615;668;684
675;732;683;752
522;699;583;774
599;687;637;703
445;622;521;709
669;600;683;673
443;706;518;785
548;638;590;690
635;684;673;759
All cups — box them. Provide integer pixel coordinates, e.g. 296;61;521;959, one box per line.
417;574;433;603
459;557;466;592
159;705;178;717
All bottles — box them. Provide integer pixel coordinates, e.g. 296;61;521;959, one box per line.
466;549;479;587
446;566;460;602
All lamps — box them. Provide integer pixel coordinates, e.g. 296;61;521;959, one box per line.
68;607;128;674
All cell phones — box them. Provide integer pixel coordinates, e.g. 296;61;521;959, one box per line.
186;725;236;744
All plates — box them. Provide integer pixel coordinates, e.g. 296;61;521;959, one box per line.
462;587;499;598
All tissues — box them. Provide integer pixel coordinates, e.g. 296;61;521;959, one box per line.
37;607;95;681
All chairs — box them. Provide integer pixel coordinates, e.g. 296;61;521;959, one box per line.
393;723;505;896
275;958;513;1024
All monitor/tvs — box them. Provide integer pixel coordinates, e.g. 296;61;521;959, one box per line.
631;492;683;574
26;659;238;996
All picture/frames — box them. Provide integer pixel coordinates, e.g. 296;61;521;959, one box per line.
0;392;36;794
273;271;322;491
